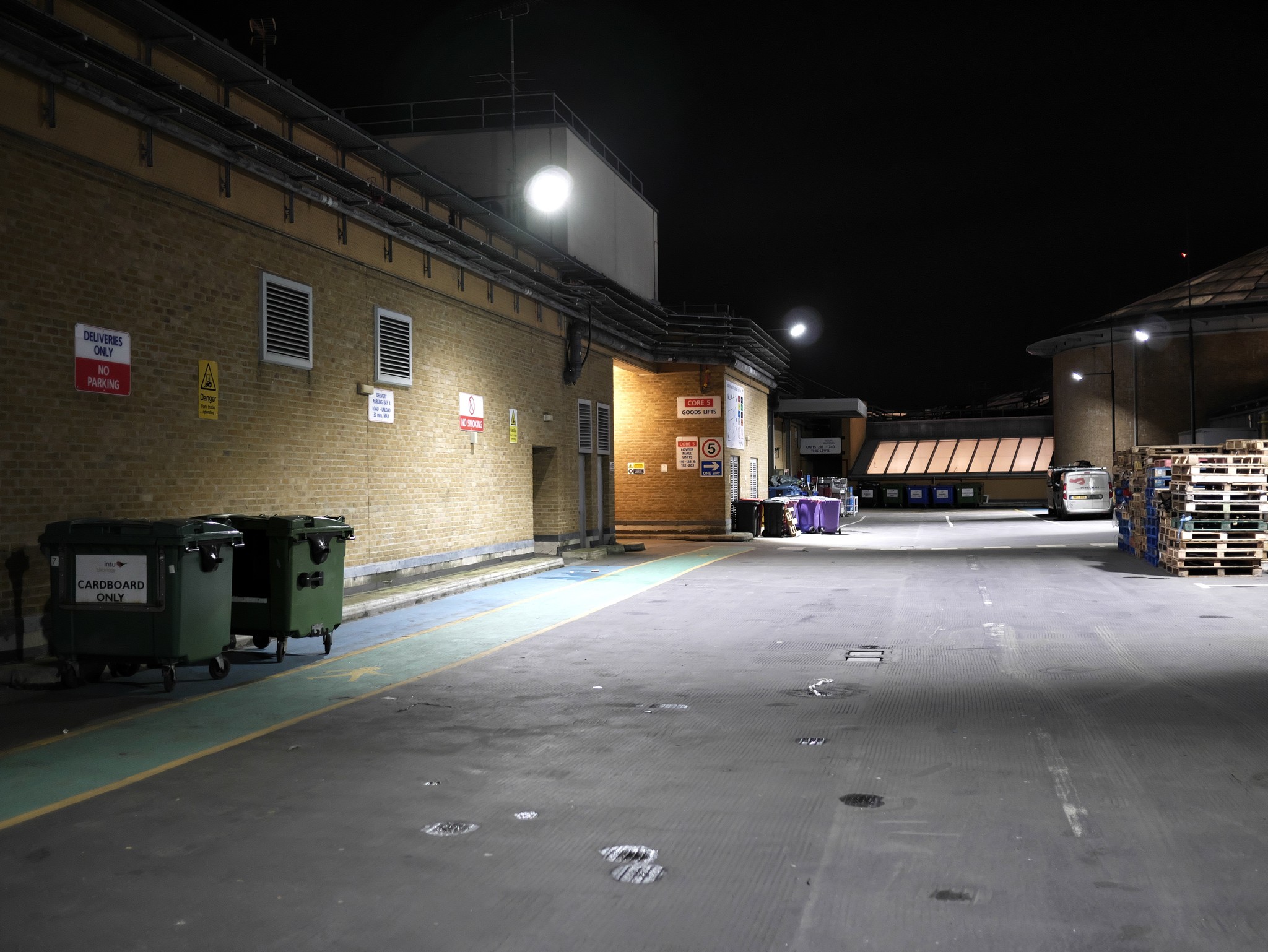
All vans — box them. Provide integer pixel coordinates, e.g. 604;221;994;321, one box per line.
1047;466;1107;515
1053;471;1113;521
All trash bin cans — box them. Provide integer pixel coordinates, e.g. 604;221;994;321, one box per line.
906;486;931;507
881;483;908;509
731;486;842;538
38;516;246;691
188;512;355;662
857;480;881;508
930;485;956;507
954;483;982;508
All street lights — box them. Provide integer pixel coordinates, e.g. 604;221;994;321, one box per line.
1071;310;1115;467
1131;329;1149;446
